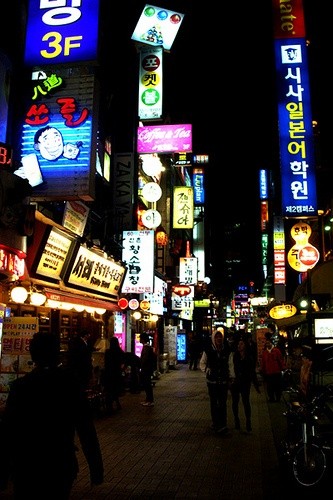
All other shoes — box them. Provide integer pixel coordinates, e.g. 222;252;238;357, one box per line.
140;402;145;404
143;402;154;406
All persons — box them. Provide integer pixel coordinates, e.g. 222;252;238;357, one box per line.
261;339;285;404
198;330;235;435
0;332;104;500
104;337;126;415
186;331;199;371
139;333;154;407
68;329;93;392
230;339;261;432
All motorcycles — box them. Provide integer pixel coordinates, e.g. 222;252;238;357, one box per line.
277;383;333;490
